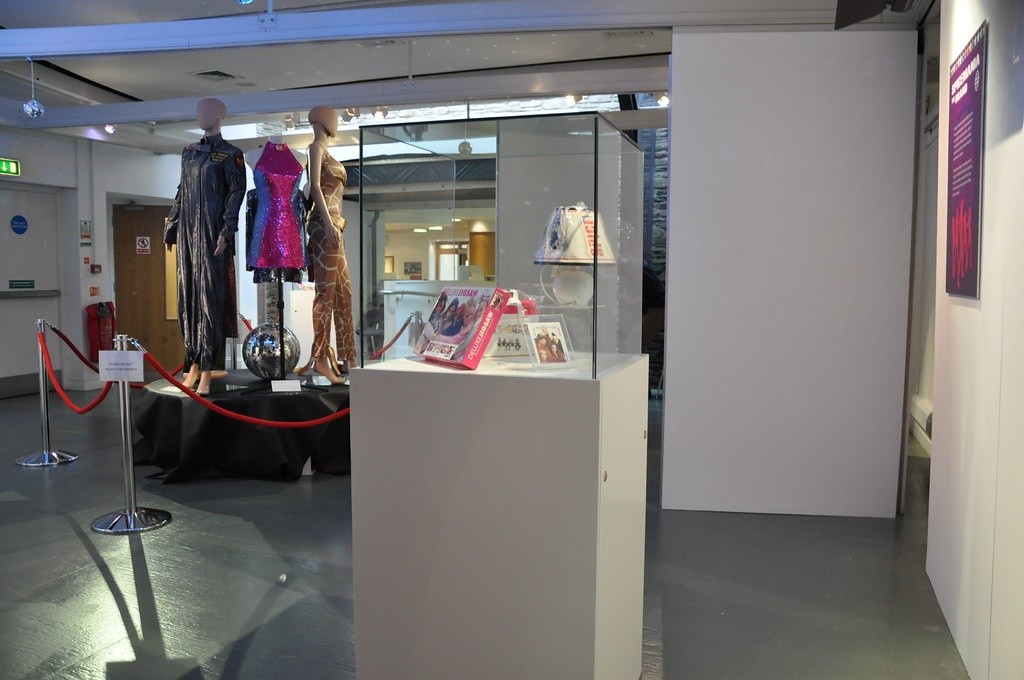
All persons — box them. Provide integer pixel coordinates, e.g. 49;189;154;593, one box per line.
246;136;307;382
162;98;246;394
534;328;566;363
304;106;358;382
416;288;490;353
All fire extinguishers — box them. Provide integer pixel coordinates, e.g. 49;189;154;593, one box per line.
95;301;113;350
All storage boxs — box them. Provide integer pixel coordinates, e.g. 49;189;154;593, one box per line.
357;110;644;379
409;286;510;370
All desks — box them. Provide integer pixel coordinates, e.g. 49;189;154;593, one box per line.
132;366;351;483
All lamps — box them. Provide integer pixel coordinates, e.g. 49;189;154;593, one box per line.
341;108;361;126
652;90;671;106
284;112;302;131
565;94;583;106
104;123;118;134
374;105;390;120
